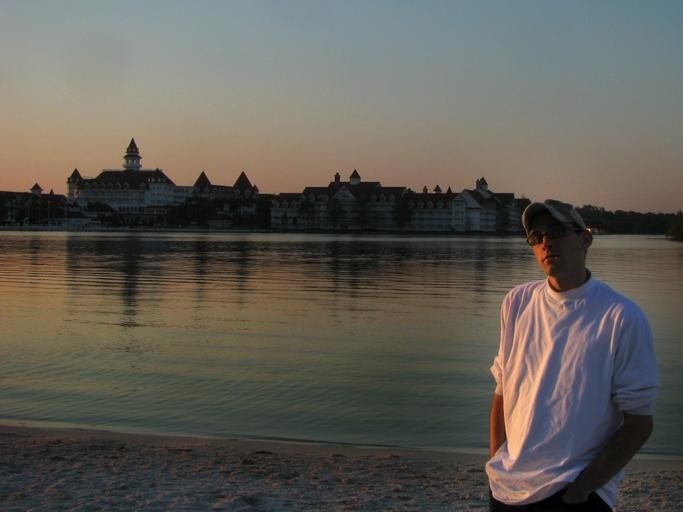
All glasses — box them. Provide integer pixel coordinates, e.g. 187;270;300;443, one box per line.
527;223;581;246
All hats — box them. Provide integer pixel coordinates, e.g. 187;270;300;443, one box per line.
522;199;587;232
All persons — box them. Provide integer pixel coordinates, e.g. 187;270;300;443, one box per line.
483;198;659;511
